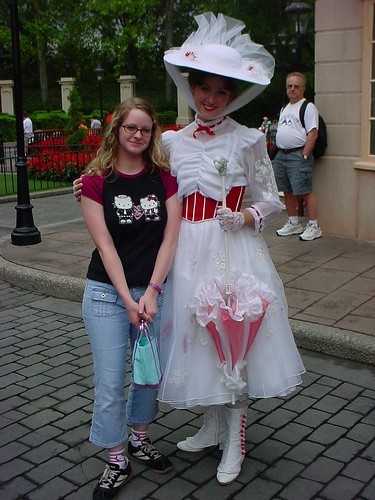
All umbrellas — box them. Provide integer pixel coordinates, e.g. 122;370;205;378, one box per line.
189;156;278;404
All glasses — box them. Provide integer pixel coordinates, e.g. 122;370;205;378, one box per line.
119;124;155;137
286;85;299;89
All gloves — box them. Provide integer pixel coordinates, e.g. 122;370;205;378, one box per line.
215;205;245;231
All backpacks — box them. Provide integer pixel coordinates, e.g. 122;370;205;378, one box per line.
282;100;327;159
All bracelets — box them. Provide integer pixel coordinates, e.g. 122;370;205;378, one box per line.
148;282;163;295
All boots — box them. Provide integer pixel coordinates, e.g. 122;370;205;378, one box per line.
216;406;247;486
176;405;224;452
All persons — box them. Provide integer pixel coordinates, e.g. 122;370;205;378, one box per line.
22;113;34;155
80;97;183;500
73;12;311;485
80;110;113;136
274;72;323;241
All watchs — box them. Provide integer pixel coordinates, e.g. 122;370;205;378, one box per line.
303;154;308;159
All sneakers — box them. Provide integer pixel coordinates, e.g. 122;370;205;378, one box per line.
298;222;322;241
93;455;134;500
127;433;175;474
275;219;303;236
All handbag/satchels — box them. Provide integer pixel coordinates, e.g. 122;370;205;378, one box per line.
130;319;163;389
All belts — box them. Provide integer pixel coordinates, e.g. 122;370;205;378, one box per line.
282;146;304;153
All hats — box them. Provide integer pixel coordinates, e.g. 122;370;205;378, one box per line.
161;44;272;86
